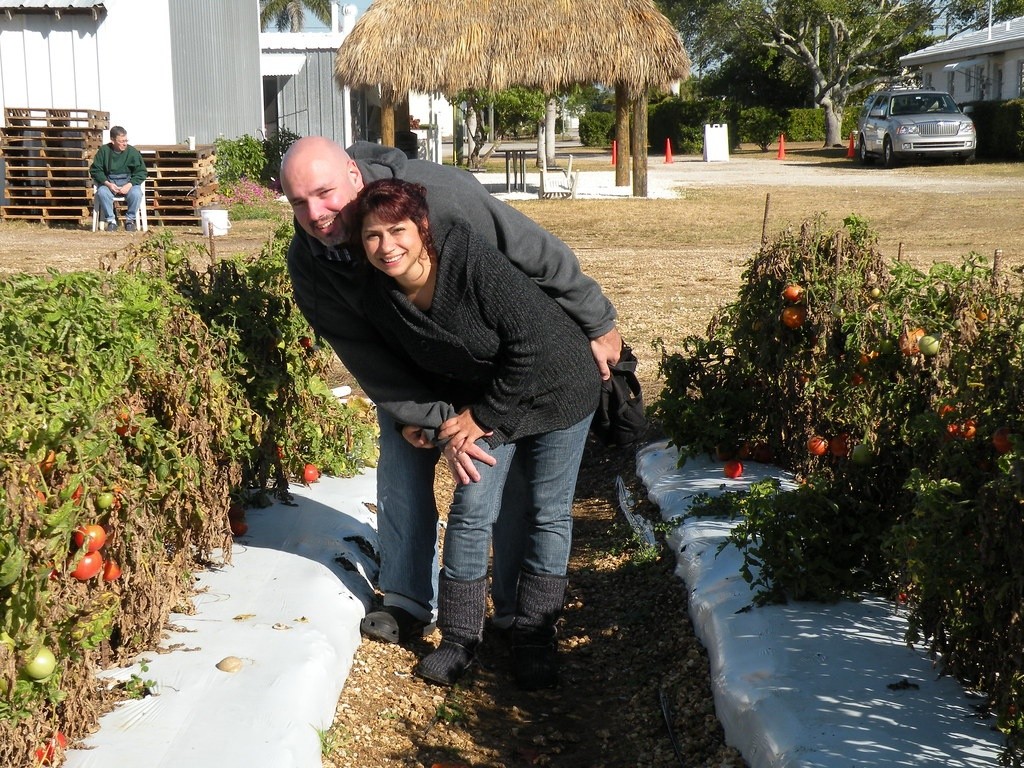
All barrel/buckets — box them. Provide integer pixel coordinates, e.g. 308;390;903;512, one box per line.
201;210;232;236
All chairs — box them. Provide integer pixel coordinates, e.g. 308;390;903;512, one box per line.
539;154;580;199
92;180;148;232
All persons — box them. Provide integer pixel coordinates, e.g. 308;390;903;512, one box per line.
89;126;148;232
356;176;602;686
280;135;624;644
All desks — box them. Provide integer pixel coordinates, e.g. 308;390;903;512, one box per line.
495;148;538;193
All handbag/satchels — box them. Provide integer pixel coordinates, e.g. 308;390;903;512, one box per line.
590;337;650;450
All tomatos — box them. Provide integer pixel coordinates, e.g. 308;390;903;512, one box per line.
0;245;323;763
713;284;1022;717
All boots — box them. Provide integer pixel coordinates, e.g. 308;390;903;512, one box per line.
418;568;490;685
507;571;569;688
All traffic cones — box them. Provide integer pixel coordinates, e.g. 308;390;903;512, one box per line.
663;137;673;164
776;134;787;159
848;132;855;157
612;140;617;165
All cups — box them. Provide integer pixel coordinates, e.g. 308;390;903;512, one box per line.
188;136;195;150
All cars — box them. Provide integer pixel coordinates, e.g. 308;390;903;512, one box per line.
858;91;976;168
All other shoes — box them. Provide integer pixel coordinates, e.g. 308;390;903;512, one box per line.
126;223;134;231
106;223;117;231
360;605;427;642
